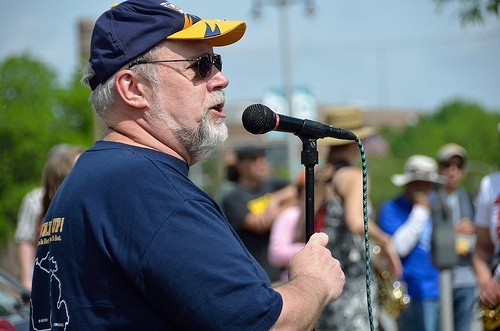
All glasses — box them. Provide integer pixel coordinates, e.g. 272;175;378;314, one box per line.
128;54;222;79
440;159;464;170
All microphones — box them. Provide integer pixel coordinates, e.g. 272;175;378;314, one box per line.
241;104;357;141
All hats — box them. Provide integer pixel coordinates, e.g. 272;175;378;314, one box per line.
88;0;246;91
439;144;467;161
392;155;448;186
317;106;375;145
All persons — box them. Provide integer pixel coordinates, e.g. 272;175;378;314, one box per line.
30;0;347;331
18;142;91;290
217;107;500;331
